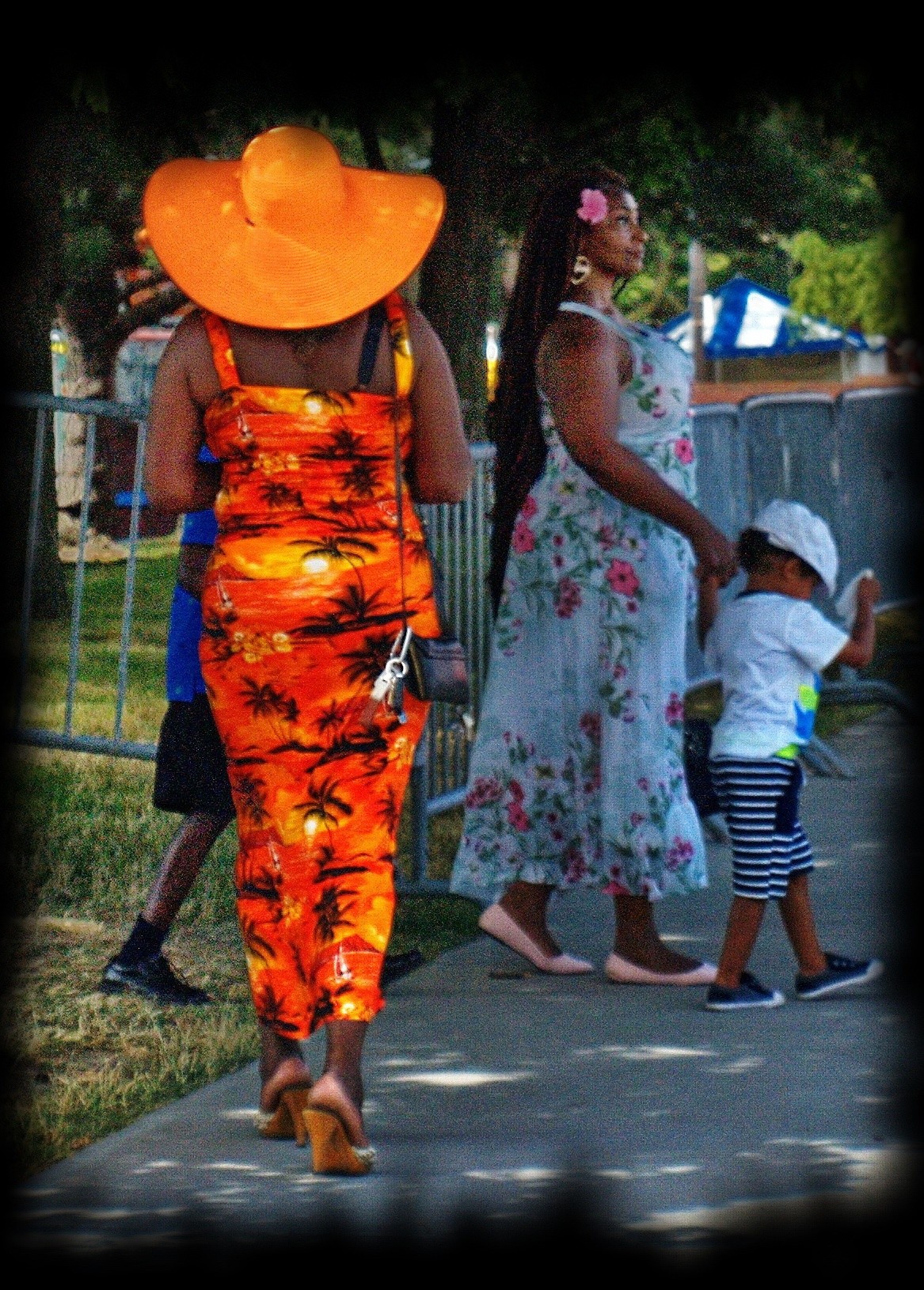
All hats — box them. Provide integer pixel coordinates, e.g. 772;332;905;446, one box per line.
143;126;445;329
750;499;839;601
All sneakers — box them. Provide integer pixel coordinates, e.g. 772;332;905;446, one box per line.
794;949;887;1002
96;954;210;1006
705;969;788;1012
383;949;424;981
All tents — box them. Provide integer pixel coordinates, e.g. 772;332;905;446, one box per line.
657;278;889;384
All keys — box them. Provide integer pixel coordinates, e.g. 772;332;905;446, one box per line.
359;653;408;734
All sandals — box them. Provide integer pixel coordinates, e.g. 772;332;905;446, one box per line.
477;903;594;975
604;952;719;985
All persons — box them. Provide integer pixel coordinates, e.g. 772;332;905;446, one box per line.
141;126;475;1177
99;435;430;1006
448;160;886;1010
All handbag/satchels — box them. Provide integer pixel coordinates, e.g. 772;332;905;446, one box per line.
402;631;471;704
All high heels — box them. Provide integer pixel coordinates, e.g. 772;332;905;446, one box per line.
302;1110;376;1176
252;1086;308;1146
